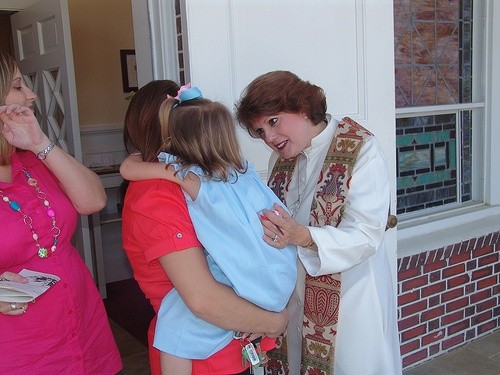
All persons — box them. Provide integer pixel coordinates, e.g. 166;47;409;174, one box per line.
120;97;297;375
119;80;290;375
0;48;123;375
237;70;402;374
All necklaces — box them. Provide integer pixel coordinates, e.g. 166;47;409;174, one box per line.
1;162;61;259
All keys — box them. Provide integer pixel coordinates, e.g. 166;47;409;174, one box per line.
234;330;267;369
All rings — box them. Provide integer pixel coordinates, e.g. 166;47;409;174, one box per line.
21;305;26;312
10;303;16;309
274;234;278;241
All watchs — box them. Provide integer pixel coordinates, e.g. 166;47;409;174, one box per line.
35;140;55;160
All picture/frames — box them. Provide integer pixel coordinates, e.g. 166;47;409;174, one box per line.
119;49;138;93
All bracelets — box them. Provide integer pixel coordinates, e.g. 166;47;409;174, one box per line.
302;241;314;248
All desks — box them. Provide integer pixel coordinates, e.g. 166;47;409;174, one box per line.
85;164;126;300
